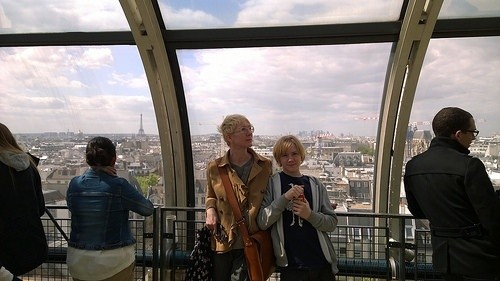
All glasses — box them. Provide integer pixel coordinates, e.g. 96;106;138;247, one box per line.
458;130;479;137
230;126;255;134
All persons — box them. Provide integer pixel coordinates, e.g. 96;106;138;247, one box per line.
0;123;49;276
403;107;500;281
205;114;272;281
65;136;154;281
257;134;339;281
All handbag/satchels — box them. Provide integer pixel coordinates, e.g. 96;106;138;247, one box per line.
244;228;273;281
